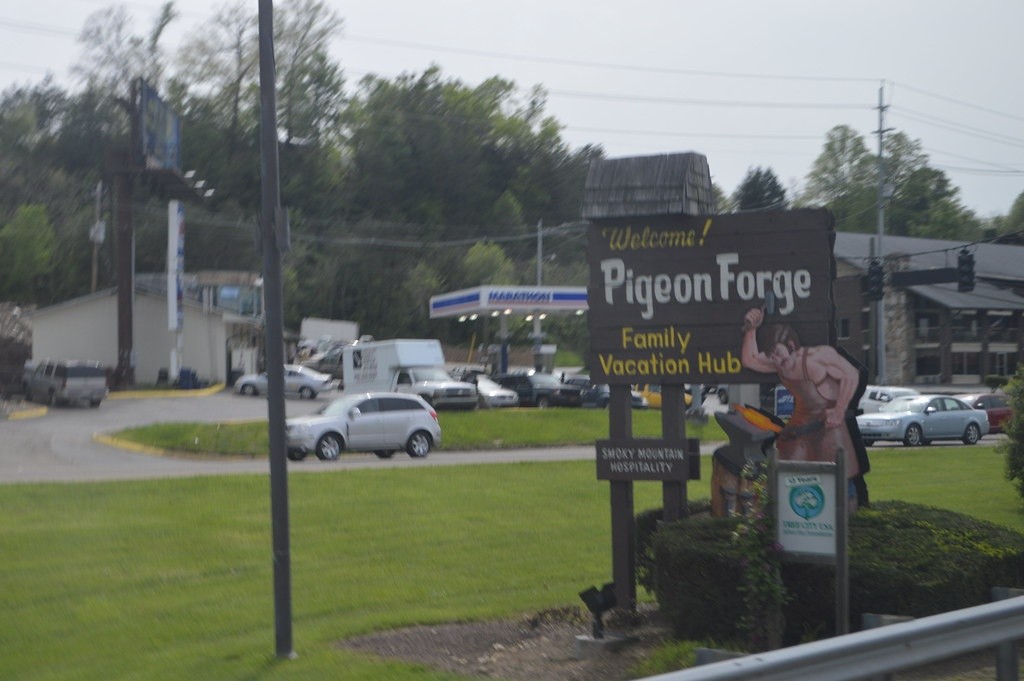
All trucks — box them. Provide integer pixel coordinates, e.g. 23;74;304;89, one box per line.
341;338;479;412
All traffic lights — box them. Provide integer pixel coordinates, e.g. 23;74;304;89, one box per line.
867;262;886;300
957;252;976;293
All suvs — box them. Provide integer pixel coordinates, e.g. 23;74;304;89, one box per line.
26;355;110;411
285;391;443;464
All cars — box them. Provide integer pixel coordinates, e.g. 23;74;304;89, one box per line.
233;363;333;403
855;393;991;448
953;392;1014;436
485;360;921;447
